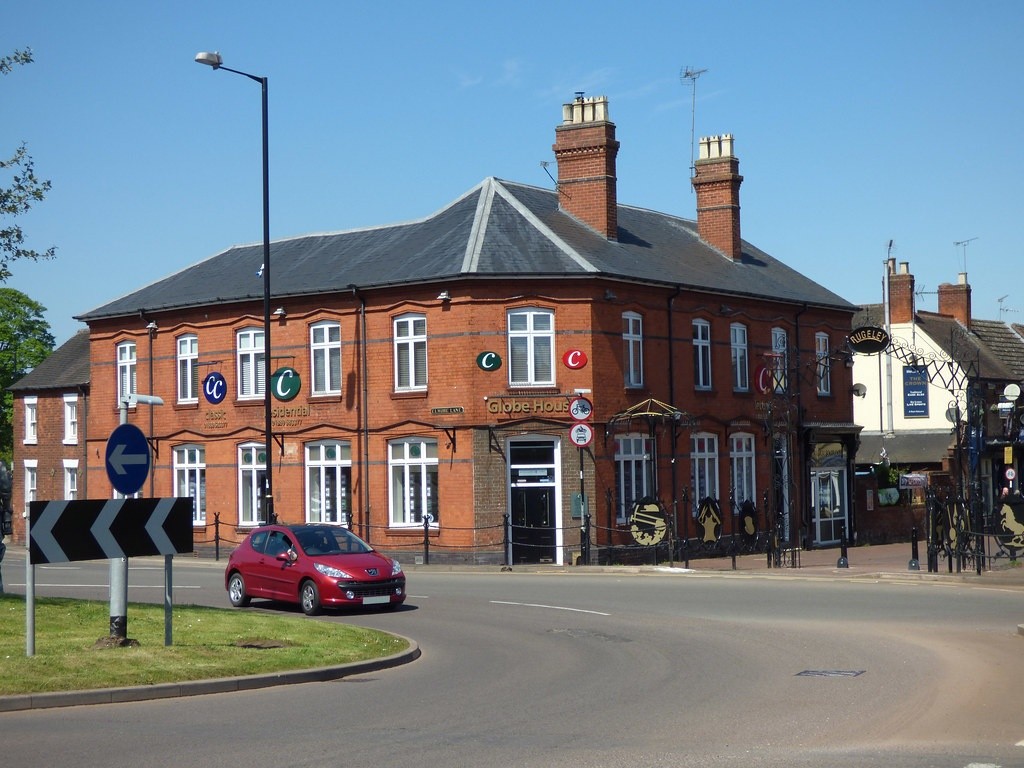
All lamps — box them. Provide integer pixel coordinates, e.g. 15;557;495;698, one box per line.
601;290;617;301
719;302;732;313
273;306;287;317
436;291;452;301
146;322;159;332
845;357;854;368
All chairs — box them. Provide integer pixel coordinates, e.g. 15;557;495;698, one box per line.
279;536;293;550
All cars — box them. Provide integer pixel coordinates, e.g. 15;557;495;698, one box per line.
224;525;406;617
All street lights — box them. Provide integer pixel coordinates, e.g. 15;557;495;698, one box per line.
192;51;278;527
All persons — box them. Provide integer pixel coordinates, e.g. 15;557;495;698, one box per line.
1000;487;1020;500
